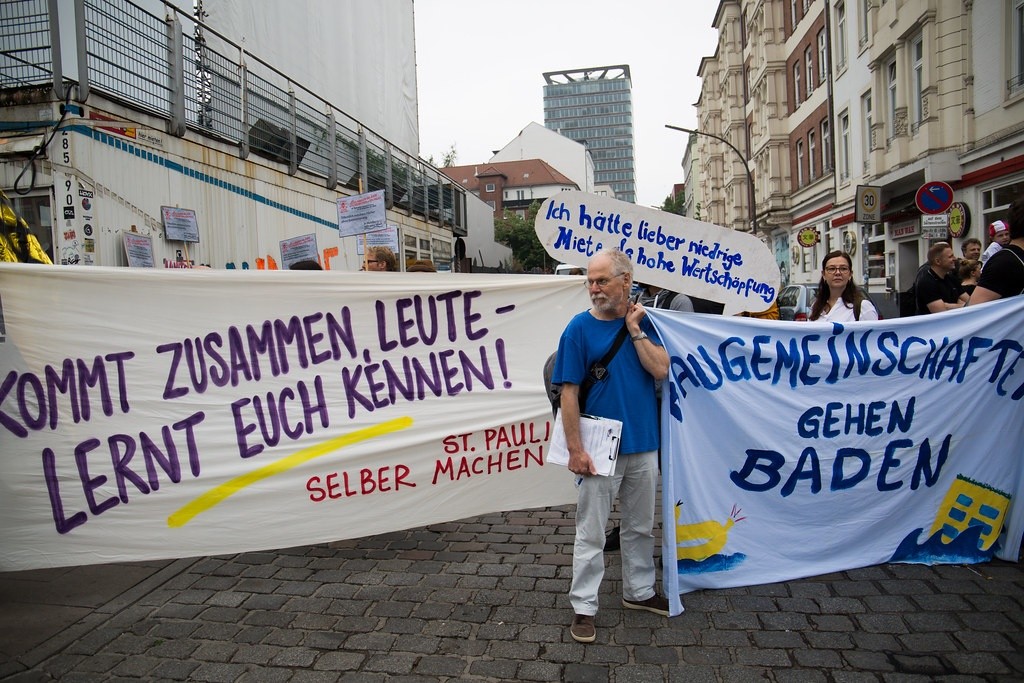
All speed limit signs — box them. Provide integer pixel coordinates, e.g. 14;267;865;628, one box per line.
854;185;881;224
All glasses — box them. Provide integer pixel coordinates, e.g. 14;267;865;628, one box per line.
584;272;626;288
825;265;848;274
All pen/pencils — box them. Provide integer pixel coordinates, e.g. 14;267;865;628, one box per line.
576;475;584;487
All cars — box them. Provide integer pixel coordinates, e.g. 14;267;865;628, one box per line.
555;265;587;275
777;284;823;322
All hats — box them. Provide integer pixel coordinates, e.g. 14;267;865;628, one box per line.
989;221;1010;236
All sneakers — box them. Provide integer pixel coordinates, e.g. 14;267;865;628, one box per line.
622;593;669;615
570;613;595;641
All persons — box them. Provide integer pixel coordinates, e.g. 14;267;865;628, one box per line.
552;247;669;642
914;244;970;314
736;299;779;319
603;283;694;570
957;257;983;296
289;260;323;270
967;192;1024;304
361;245;397;272
963;239;982;261
568;268;582;275
982;219;1010;270
808;250;878;321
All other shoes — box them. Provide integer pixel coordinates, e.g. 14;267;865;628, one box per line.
604;526;620;551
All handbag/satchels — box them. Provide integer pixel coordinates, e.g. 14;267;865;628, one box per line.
544;322;629;420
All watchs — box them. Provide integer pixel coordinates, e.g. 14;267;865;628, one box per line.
630;331;647;343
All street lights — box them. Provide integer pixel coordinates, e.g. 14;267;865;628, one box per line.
665;124;758;237
